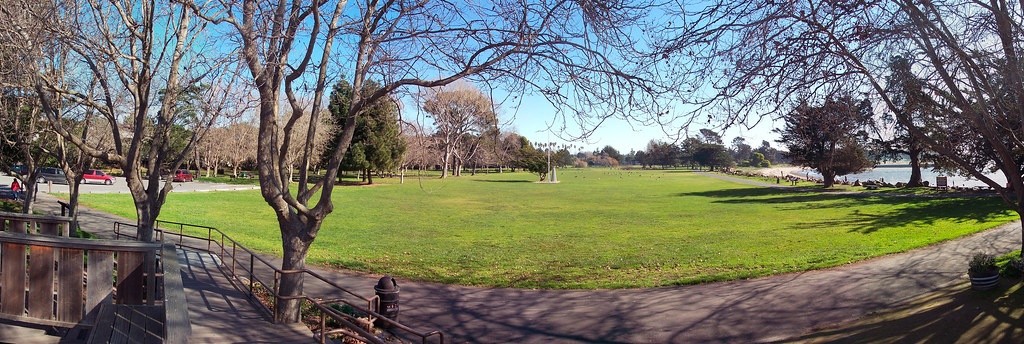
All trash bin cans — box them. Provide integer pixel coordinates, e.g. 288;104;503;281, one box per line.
374;274;400;329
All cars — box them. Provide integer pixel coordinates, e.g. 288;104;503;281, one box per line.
35;166;70;185
80;169;116;185
0;176;27;202
11;164;28;175
173;169;195;183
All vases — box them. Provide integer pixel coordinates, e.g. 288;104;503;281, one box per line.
969;273;1000;292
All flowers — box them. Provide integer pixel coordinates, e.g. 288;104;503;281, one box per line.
968;253;995;274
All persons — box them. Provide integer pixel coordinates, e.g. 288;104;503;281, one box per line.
952;180;954;187
806;172;809;182
875;180;880;183
11;178;22;201
845;176;847;182
866;178;871;183
854;179;861;186
881;176;884;183
781;171;784;179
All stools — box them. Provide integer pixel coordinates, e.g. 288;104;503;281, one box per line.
87;303;164;344
